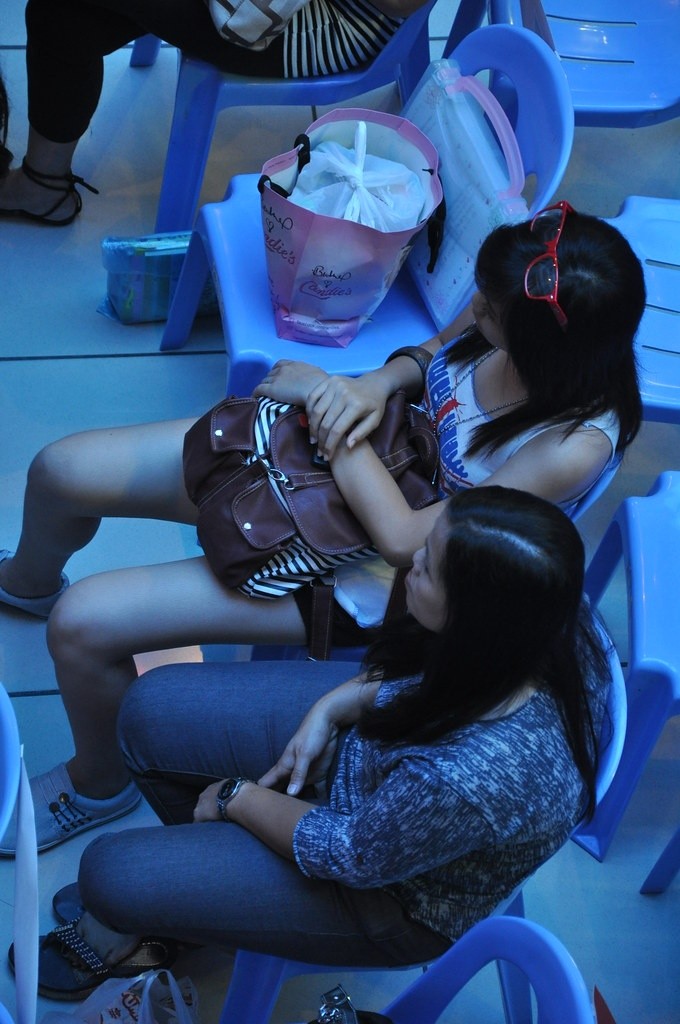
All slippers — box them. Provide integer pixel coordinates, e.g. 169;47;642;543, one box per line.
8;918;179;1002
52;881;153;925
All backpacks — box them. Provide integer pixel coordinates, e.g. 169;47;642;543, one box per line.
183;346;441;600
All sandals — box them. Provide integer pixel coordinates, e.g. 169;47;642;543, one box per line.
2;155;84;228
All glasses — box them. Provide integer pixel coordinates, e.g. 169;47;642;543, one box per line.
523;198;574;325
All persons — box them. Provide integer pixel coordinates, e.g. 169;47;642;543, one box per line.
0;2;420;231
11;483;608;1003
1;207;646;864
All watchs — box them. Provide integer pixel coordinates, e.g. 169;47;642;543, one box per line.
215;775;258;824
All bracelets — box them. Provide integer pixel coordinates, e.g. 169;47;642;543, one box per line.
386;345;434;388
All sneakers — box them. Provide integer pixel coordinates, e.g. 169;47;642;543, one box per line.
1;760;141;857
0;551;72;619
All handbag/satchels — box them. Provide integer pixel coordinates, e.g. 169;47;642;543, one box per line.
401;58;539;341
259;107;442;349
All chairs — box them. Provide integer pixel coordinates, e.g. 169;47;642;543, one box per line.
0;0;680;1024
